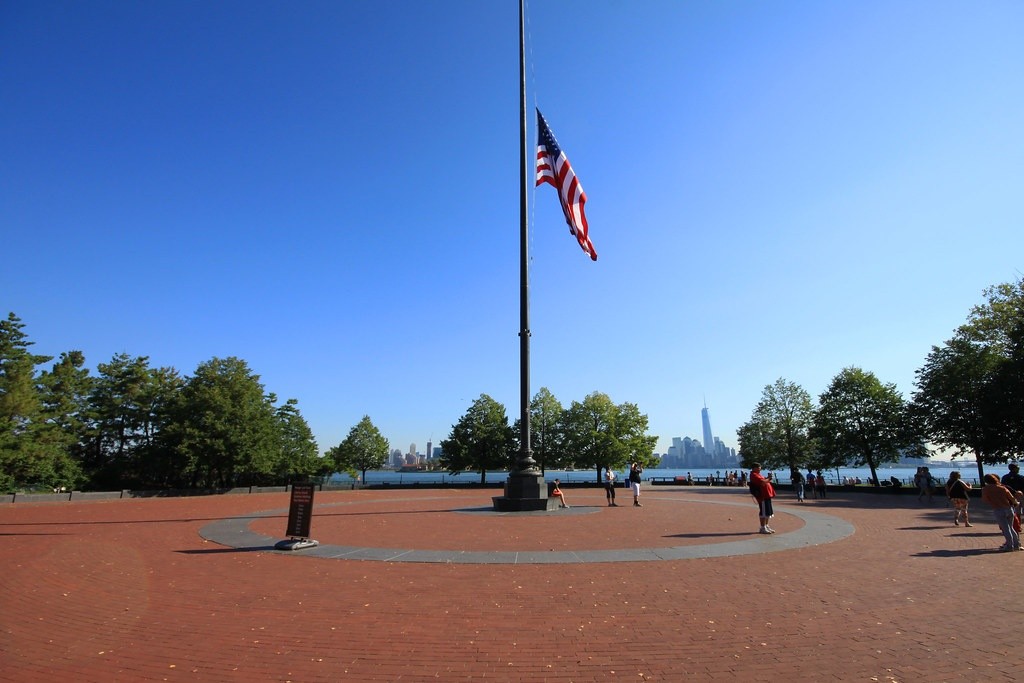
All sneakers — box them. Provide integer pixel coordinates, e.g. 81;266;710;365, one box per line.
760;527;771;534
766;526;775;533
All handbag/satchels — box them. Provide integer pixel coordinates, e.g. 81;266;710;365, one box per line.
749;473;761;497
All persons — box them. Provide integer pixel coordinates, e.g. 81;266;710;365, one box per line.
630;462;644;507
548;479;570;508
688;463;1024;552
750;462;777;534
604;465;619;507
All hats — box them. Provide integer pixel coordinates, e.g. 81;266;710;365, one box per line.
751;462;761;469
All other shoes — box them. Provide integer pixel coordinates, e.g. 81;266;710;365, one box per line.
612;503;618;506
562;505;569;508
955;519;959;526
559;505;561;507
965;522;973;527
633;502;643;507
998;540;1024;552
609;503;614;507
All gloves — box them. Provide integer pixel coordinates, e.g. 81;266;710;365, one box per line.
768;470;774;480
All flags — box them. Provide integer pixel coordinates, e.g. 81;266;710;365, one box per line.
535;105;598;262
